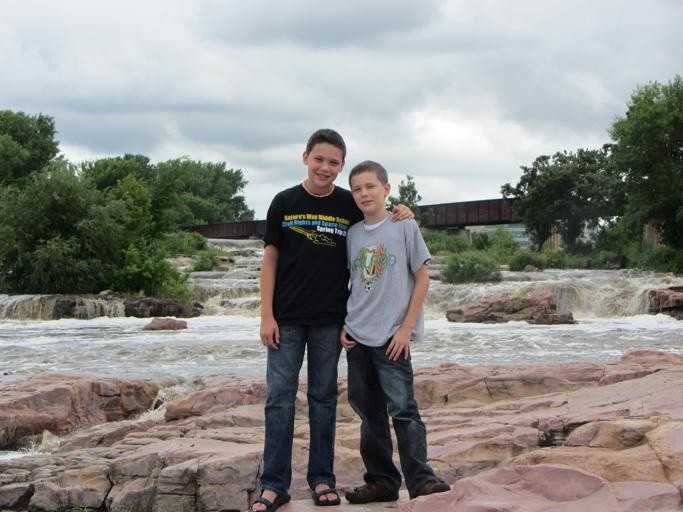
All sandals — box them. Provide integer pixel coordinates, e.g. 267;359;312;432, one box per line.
251;482;291;512
313;482;343;507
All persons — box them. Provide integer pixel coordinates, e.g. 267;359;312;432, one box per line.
337;159;450;504
248;128;415;512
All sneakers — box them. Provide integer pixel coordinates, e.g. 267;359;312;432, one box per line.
344;479;401;504
408;477;451;501
